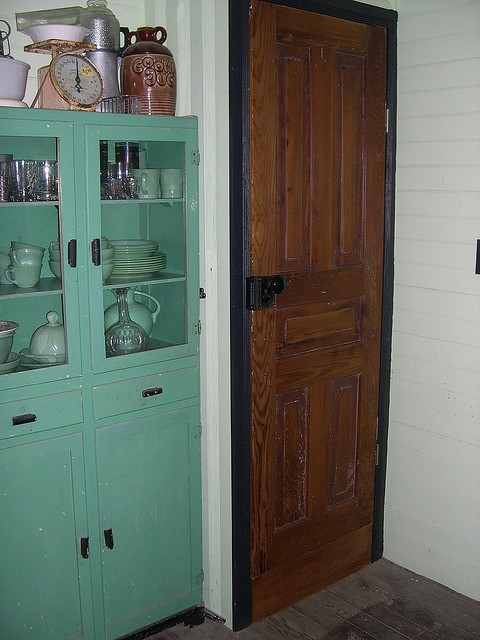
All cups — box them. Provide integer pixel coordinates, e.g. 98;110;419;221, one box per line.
100;162;140;200
10;240;46;253
0;154;58;202
133;168;160;199
0;246;12;265
0;265;13;285
5;264;43;288
8;248;44;267
160;169;183;198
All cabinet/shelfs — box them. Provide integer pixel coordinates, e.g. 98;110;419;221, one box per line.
0;106;202;640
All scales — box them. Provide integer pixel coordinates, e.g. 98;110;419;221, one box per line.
25;39;103;112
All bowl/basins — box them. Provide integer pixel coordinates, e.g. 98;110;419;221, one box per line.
0;352;20;375
49;236;115;284
22;24;93;44
0;332;16;364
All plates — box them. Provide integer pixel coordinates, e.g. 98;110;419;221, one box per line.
19;348;67;364
0;321;19;334
18;358;66;370
108;240;167;278
